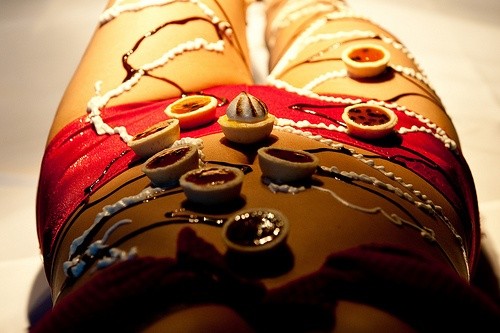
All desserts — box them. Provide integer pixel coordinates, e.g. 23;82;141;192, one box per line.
39;0;480;333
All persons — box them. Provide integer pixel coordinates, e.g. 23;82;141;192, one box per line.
37;0;498;332
